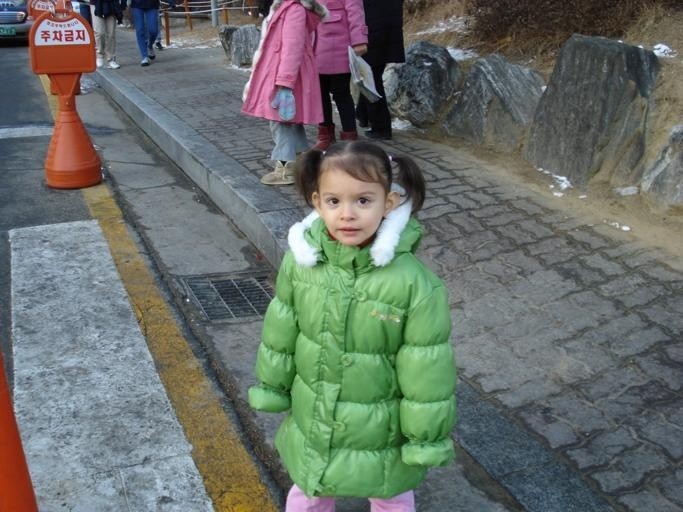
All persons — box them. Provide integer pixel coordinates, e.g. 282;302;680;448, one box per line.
247;138;460;512
90;1;127;69
314;0;368;150
356;2;406;139
130;0;161;66
241;0;328;187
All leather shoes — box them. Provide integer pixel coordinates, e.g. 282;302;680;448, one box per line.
359;119;368;127
365;130;391;141
155;42;163;50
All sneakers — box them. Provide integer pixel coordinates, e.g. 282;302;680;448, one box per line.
140;57;150;66
147;48;155;60
95;58;104;68
107;60;120;69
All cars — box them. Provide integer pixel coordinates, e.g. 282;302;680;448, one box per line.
0;0;35;39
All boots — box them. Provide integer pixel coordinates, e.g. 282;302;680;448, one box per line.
260;158;298;185
312;125;335;153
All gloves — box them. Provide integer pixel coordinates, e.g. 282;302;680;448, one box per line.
270;86;296;122
